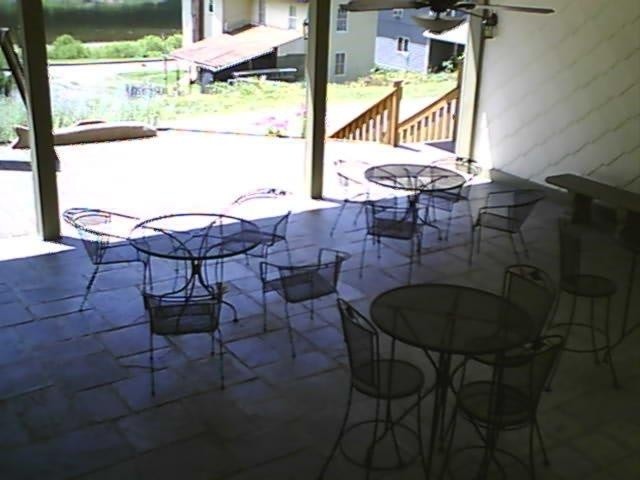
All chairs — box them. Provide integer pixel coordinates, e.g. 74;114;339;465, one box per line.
256;244;353;358
328;157;378;244
549;213;621;394
213;188;298;297
138;280;233;399
421;155;480;244
457;262;559;417
318;296;428;478
467;186;547;266
356;199;426;288
61;206;156;320
446;332;569;480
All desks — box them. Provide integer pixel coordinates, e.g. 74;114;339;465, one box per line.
363;161;467;260
125;211;266;334
368;280;539;460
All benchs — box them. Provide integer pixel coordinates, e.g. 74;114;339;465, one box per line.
546;170;638;249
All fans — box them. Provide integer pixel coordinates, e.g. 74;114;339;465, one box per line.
336;0;561;22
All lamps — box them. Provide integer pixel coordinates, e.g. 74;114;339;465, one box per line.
412;11;469;37
479;12;500;39
